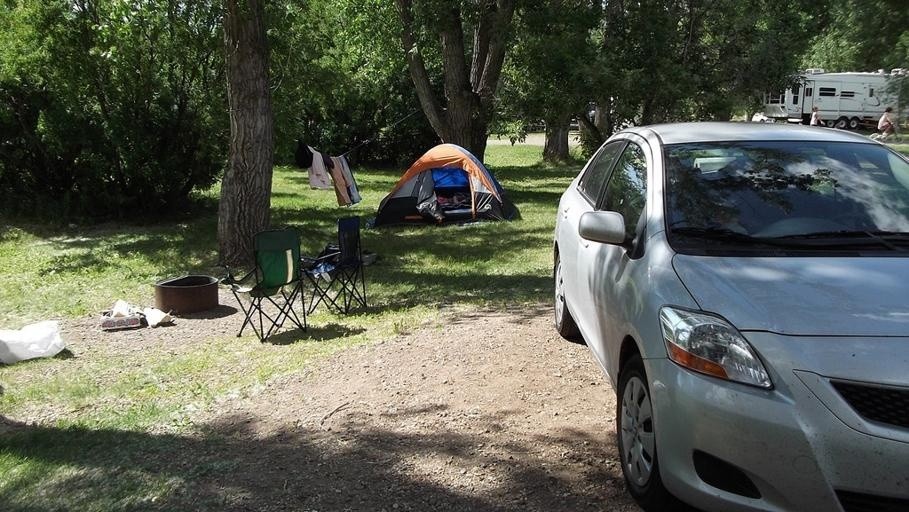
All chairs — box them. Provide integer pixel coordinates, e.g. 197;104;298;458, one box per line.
300;216;367;316
228;228;308;344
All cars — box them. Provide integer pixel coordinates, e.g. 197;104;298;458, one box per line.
551;120;909;512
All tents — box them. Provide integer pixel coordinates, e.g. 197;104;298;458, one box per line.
372;142;523;228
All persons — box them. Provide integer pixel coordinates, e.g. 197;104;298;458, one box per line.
810;106;828;127
878;107;898;139
